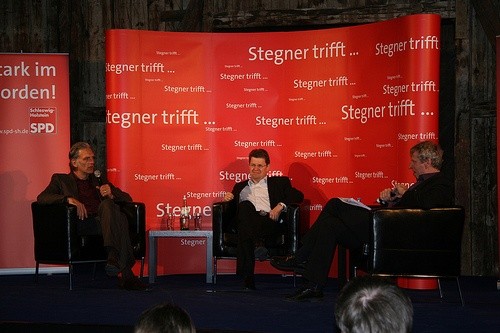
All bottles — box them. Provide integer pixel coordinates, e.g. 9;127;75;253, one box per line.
180;195;189;230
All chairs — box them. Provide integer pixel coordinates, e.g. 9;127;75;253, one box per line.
32;201;146;290
212;203;300;288
338;204;465;307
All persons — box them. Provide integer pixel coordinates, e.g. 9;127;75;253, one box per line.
270;141;449;303
222;149;304;289
37;142;137;289
134;303;196;333
334;277;412;333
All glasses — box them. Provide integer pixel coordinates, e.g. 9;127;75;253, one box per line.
248;163;266;169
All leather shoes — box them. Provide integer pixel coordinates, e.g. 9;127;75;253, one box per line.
105;261;132;284
270;252;308;277
279;286;324;304
239;278;256;293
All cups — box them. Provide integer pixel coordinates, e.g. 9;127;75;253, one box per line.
195;213;202;230
166;214;174;230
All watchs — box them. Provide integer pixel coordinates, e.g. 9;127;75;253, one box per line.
278;203;285;210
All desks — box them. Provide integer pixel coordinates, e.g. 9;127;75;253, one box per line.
149;229;213;283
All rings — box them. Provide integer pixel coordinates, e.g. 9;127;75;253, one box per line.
104;190;107;193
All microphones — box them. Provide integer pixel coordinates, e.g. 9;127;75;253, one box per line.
94;170;104;187
259;210;283;224
375;188;400;204
